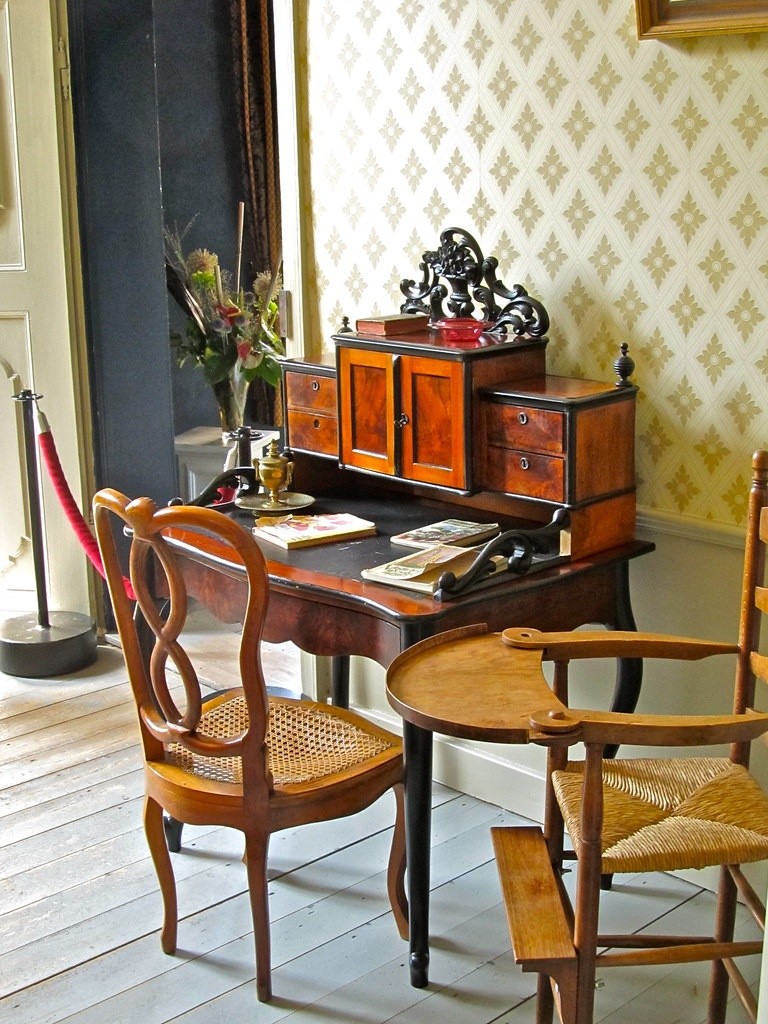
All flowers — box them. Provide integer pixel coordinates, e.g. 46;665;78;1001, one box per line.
164;202;288;431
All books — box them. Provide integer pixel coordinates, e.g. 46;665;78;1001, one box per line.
389;519;501;550
360;544;509;592
252;512;377;550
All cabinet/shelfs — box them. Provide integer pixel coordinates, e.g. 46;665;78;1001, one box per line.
280;228;642;564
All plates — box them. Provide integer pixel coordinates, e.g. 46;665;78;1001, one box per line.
235;492;315;511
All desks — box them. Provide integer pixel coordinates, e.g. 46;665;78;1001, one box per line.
173;424;279;504
385;623;583;749
122;455;656;991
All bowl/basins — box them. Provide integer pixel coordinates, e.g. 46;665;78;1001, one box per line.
438;319;485;341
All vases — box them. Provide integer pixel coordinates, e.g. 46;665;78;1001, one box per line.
197;355;250;441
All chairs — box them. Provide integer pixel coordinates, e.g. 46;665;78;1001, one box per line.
490;446;768;1024
91;487;409;1001
